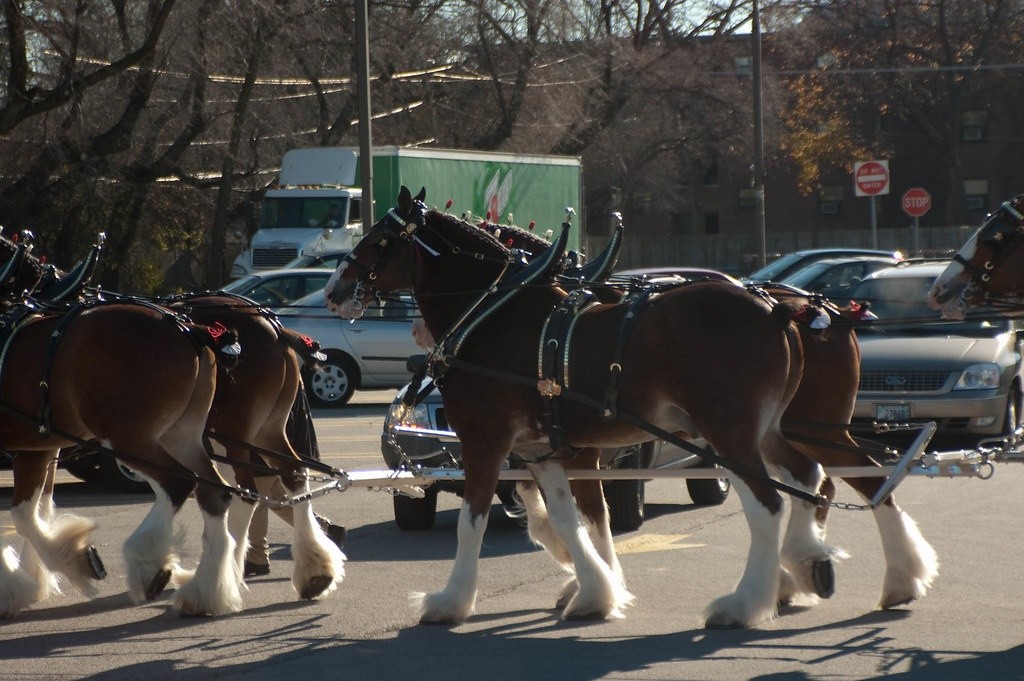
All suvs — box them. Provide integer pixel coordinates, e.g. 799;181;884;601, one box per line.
379;272;730;534
606;247;911;309
845;255;1024;450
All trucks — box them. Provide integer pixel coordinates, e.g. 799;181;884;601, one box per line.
228;146;585;304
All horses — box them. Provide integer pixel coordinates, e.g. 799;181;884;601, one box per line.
0;182;944;629
926;194;1023;323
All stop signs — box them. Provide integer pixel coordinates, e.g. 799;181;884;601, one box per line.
902;188;931;218
853;159;890;198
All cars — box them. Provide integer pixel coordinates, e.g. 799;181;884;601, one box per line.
220;267;434;410
244;244;362;311
